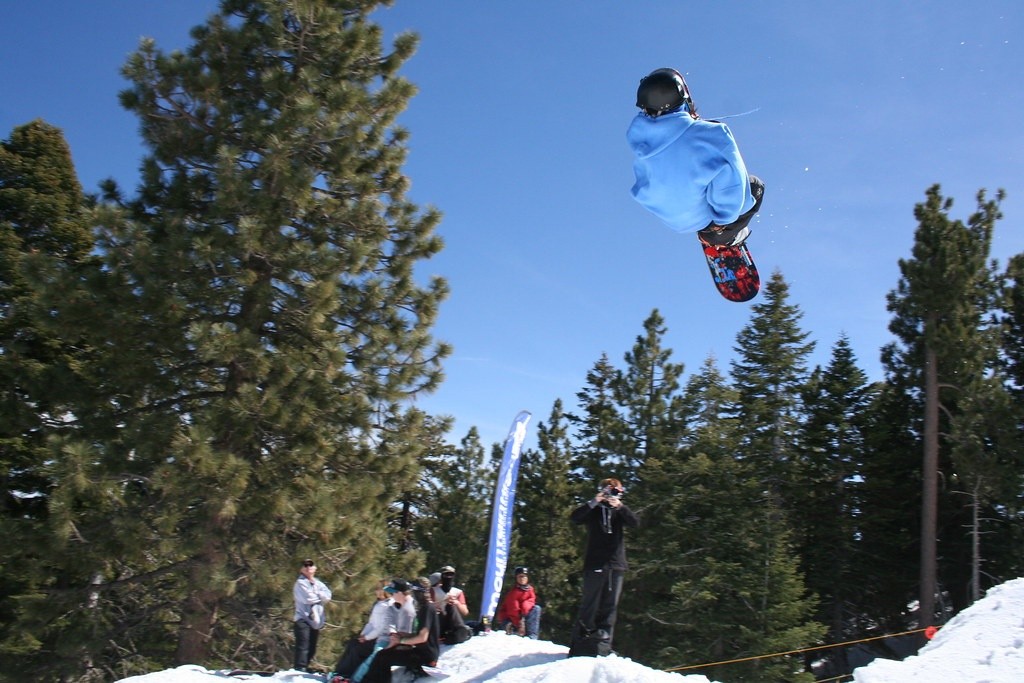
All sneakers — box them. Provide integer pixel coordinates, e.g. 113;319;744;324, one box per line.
482;615;491;633
463;625;474;636
718;229;753;249
700;225;724;245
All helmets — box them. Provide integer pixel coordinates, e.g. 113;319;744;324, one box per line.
515;566;529;575
636;67;691;112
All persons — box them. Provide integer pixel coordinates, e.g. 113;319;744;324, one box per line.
568;478;639;656
292;560;331;673
626;68;765;248
329;566;468;683
497;566;541;640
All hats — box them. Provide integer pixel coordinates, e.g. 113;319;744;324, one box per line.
430;572;442;586
442;566;456;574
383;580;409;594
302;559;314;563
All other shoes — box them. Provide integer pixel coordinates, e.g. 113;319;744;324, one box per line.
327;672;350;683
296;665;313;673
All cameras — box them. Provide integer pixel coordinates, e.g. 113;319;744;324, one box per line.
604;487;623;500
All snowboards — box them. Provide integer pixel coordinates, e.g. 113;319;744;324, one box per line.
646;68;760;302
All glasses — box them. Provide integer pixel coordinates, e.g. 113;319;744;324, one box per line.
303;563;314;567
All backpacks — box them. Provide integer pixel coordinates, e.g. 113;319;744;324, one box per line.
296;577;327;629
